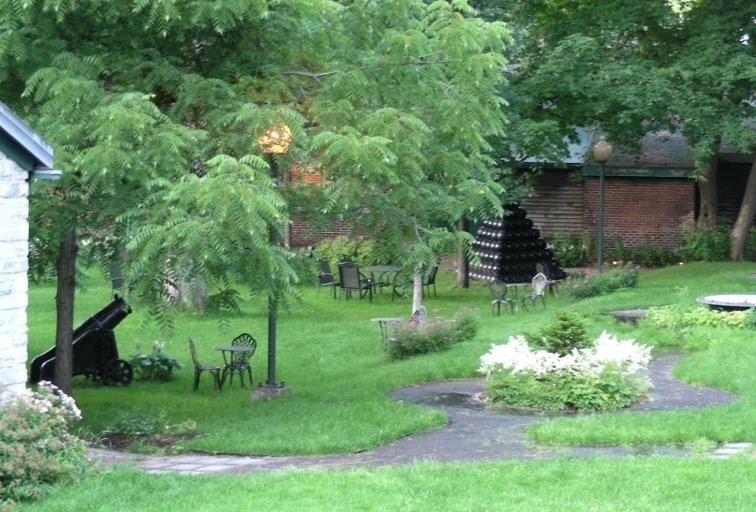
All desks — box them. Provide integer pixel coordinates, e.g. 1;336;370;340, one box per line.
505;279;558;314
216;345;252;391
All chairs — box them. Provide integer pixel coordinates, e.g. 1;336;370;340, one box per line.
316;257;438;304
189;337;223;392
489;271;547;316
230;334;257;389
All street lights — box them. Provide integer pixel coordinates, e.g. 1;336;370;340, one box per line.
592;135;613;271
